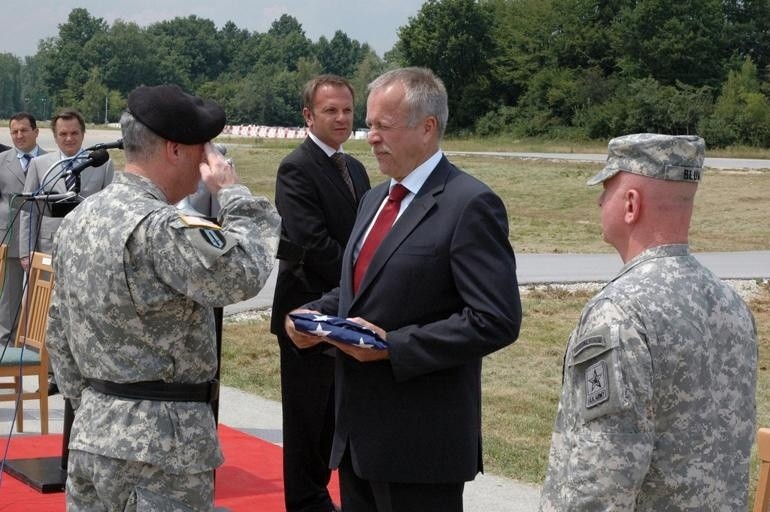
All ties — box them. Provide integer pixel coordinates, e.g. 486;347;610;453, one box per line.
64;159;76;192
333;155;355;200
23;154;31;174
350;185;407;304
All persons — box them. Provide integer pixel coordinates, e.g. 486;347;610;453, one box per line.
18;109;114;397
540;133;757;512
274;73;372;511
46;83;282;512
0;112;49;346
285;68;523;512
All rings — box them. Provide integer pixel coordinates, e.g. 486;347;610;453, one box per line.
227;159;233;165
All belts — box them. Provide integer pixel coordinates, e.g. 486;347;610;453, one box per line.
80;376;221;403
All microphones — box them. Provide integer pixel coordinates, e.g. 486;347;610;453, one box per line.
92;136;126;151
15;190;58;196
58;149;109;180
34;190;76;200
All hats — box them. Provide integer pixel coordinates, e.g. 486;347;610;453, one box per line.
128;85;225;145
585;134;707;186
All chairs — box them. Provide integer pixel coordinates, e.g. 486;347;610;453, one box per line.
0;251;56;434
